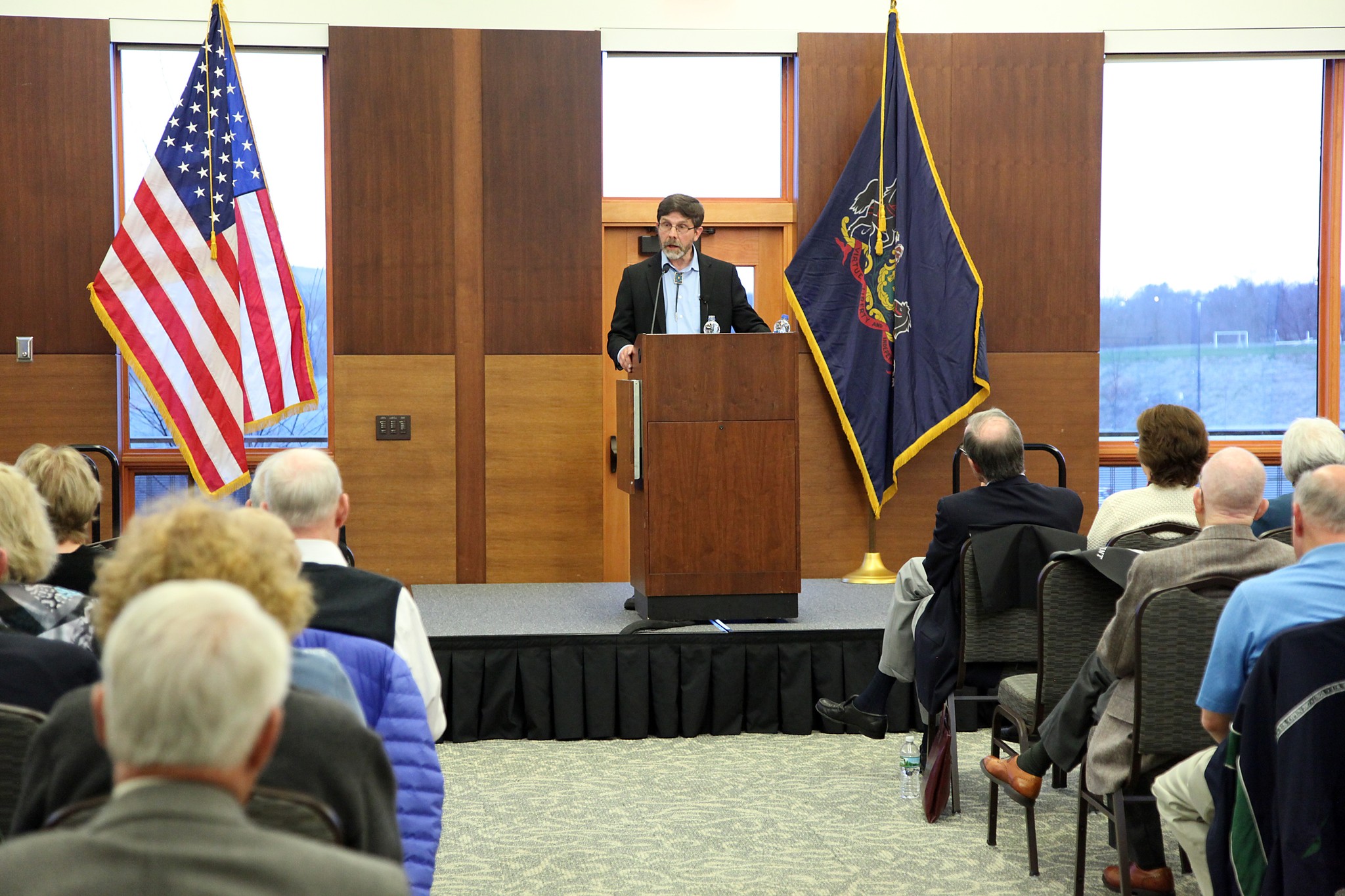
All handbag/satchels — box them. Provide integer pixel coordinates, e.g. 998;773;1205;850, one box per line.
920;703;952;823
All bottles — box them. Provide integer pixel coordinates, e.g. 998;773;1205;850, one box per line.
703;315;721;333
773;314;791;333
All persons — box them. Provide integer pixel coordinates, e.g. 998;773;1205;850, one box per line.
245;458;265;509
13;443;111;595
0;463;98;651
0;477;99;716
606;194;772;609
980;447;1298;896
260;451;447;740
1250;417;1345;539
14;494;401;859
815;407;1084;774
1152;463;1345;896
0;578;411;896
1087;404;1209;550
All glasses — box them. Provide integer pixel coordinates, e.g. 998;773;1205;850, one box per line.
659;221;697;234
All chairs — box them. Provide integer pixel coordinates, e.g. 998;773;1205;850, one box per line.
927;519;1345;896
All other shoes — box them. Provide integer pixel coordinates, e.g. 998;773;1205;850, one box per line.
1000;727;1019;744
624;594;636;610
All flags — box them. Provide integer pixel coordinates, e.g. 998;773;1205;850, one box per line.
784;10;992;519
87;0;320;498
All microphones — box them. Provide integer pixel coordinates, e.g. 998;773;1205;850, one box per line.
650;265;670;334
699;296;704;300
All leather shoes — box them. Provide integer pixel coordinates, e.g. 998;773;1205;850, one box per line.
920;743;927;770
1102;861;1176;896
815;694;888;740
980;755;1042;808
